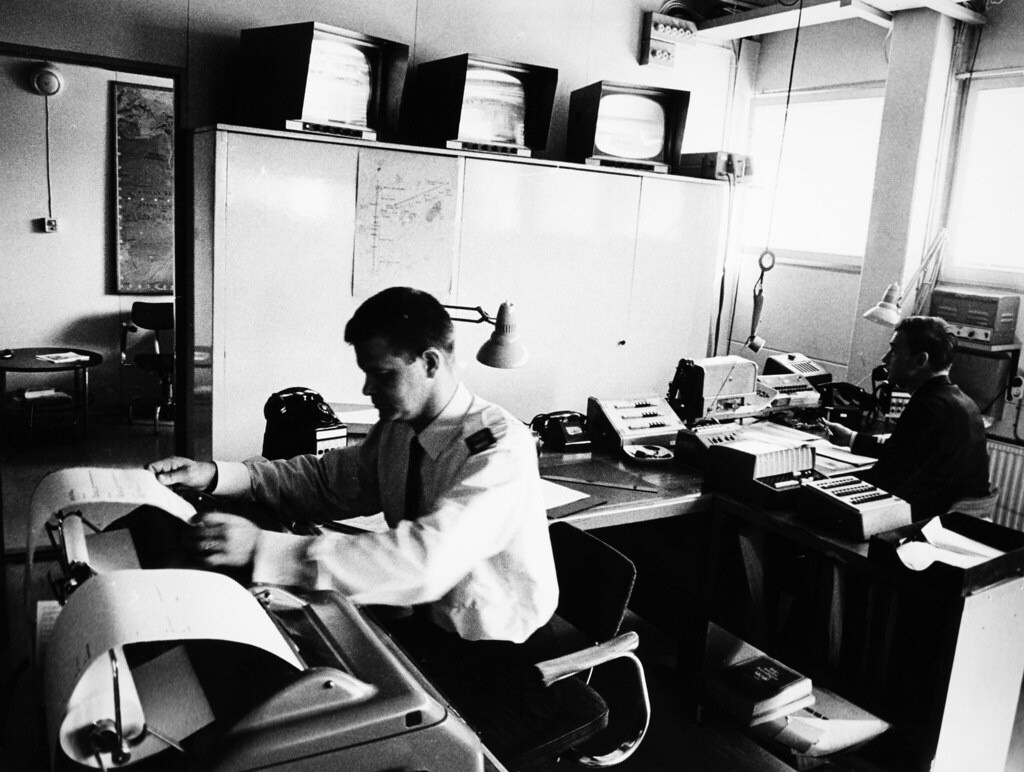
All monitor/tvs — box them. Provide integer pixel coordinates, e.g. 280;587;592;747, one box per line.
237;21;410;144
567;79;691;175
415;53;558;157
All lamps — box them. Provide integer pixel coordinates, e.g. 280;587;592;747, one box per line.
437;299;528;369
861;223;949;335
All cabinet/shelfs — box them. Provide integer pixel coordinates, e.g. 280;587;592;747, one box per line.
187;128;727;466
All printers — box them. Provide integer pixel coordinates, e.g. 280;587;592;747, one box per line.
45;480;485;772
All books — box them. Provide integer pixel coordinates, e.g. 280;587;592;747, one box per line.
36;352;90;364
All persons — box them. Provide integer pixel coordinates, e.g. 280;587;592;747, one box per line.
815;316;991;522
144;285;562;709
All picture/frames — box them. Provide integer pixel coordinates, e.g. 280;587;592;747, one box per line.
111;79;175;297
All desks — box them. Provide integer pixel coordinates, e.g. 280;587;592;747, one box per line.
0;348;102;447
36;445;1024;772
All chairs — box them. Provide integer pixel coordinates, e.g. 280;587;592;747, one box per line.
116;302;176;431
949;487;1003;524
465;523;653;772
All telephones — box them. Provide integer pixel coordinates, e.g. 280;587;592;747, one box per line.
264;385;341;430
872;363;889;381
533;409;592;454
